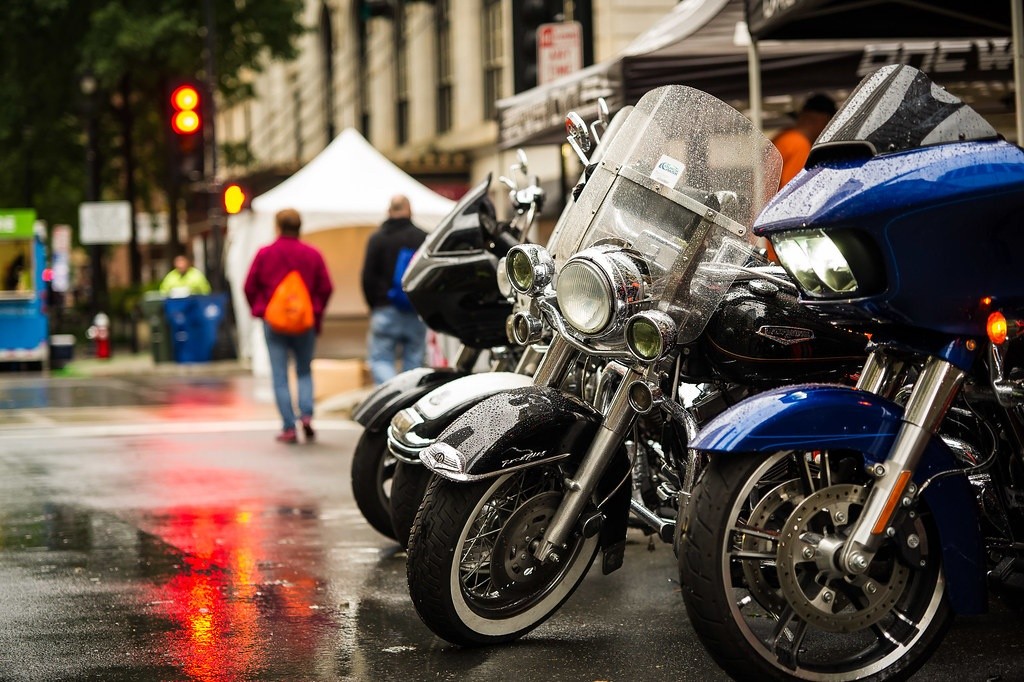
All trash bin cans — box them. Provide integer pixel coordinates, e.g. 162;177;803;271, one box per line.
140;293;226;363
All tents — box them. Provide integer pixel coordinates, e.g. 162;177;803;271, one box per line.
226;129;459;400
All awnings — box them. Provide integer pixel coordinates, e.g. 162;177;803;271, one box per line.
495;0;1024;152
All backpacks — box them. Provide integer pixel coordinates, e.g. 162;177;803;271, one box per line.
264;245;314;334
382;230;425;314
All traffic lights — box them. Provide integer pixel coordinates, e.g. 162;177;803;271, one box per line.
169;80;199;139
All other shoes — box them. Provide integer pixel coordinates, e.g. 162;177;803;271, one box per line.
301;416;314;436
277;428;297;443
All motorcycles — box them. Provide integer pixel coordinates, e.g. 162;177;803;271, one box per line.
674;64;1024;682
351;86;870;650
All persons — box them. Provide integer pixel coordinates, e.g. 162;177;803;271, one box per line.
244;209;333;442
758;93;839;265
160;255;211;298
360;198;432;385
